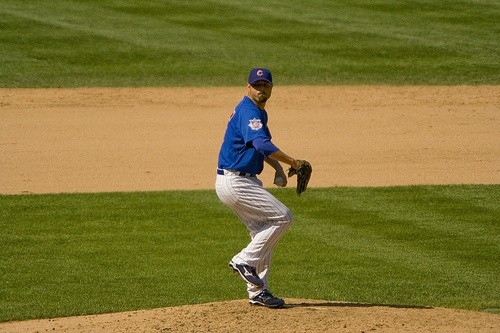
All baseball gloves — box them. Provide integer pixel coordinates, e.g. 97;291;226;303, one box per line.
288;160;312;198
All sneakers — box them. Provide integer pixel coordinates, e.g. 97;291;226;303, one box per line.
248;289;284;308
228;260;263;288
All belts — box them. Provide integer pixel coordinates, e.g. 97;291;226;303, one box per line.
216;170;254;176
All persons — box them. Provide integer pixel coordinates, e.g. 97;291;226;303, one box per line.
214;68;309;308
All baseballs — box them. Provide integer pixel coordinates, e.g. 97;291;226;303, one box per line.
277;177;283;183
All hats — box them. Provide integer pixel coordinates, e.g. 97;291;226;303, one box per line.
247;68;273;85
